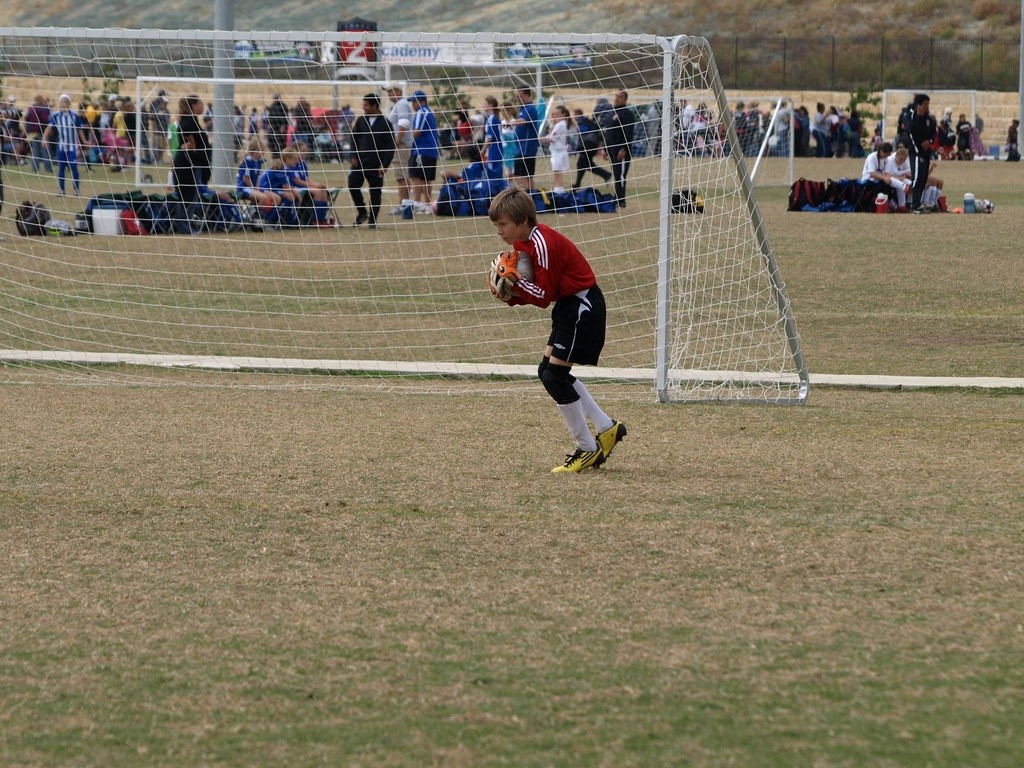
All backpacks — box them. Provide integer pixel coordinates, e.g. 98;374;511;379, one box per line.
441;174;826;216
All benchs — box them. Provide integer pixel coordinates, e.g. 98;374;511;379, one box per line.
128;186;342;238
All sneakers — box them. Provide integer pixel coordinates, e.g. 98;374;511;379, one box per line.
549;447;607;474
388;206;405;216
588;421;629;471
352;213;367;228
413;202;422;215
367;217;378;228
424;203;434;214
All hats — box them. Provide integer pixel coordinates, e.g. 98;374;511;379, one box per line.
407;91;428;101
382;82;403;94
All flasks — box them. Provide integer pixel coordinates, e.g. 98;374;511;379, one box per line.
875;194;888;212
963;193;975;212
402;199;414;219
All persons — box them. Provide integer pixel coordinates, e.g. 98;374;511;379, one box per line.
480;94;504;179
498;100;520;185
348;92;397;229
899;93;938;213
236;138;282;234
404;88;438;216
907;149;934;211
0;76;1024;174
260;147;305;231
42;94;93;197
380;79;415;215
862;142;900;214
180;94;213;184
601;90;634;208
929;161;944;212
885;146;917;213
175;133;251;231
568;108;614;191
489;188;628;475
503;85;539;192
288;140;335;231
440;144;487;199
540;105;574;190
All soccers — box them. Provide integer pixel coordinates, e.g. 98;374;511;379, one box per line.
982;200;994;213
975;199;983;213
499;250;533;295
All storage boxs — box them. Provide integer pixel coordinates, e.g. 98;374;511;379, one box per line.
92;205;124;235
